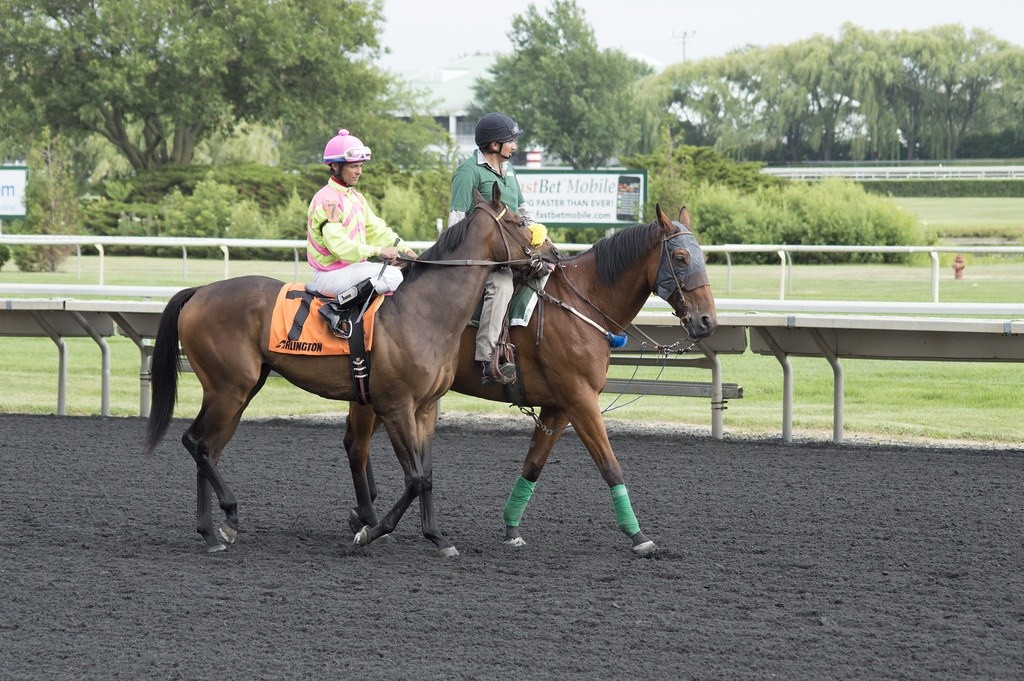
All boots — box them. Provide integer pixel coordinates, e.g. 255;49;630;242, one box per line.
318;278;379;336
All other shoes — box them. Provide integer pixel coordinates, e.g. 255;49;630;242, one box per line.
479;362;516;388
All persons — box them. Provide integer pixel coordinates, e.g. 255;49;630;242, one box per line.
306;129;419;335
448;112;547;384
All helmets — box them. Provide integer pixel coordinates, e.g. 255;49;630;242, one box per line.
475;112;523;148
322;129;371;164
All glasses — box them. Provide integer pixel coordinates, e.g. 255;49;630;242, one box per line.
322;146;371;161
511;122;520;137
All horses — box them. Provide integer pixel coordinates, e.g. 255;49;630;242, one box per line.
141;180;718;559
